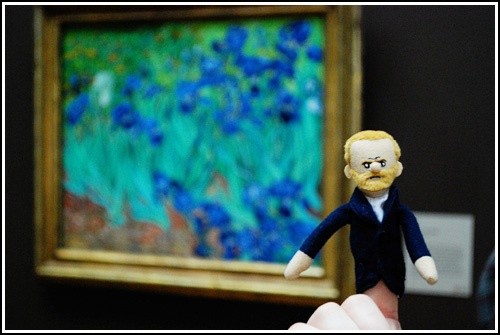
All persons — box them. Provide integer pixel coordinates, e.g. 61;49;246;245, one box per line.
282;128;440;329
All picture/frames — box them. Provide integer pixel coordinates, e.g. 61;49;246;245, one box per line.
33;5;362;309
